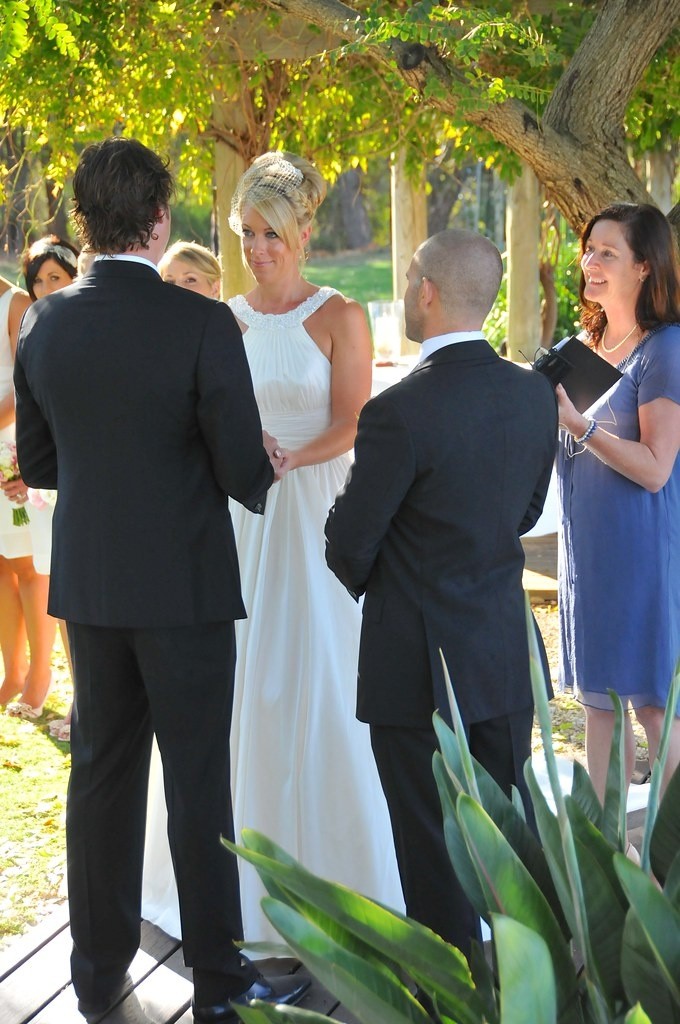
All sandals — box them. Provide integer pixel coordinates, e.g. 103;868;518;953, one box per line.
48;719;71;741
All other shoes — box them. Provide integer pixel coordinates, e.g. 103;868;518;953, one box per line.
627;843;641;865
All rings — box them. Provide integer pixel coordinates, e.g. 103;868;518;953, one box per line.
17;494;26;498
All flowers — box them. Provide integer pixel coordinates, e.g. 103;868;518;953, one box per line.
0;441;29;527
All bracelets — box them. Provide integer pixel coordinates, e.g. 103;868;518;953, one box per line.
574;420;597;445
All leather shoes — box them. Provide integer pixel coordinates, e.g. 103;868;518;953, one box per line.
78;996;111;1013
192;975;312;1024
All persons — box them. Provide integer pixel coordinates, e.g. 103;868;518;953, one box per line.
12;138;312;1024
325;227;574;1020
157;241;220;299
558;204;680;872
142;149;405;946
0;237;96;742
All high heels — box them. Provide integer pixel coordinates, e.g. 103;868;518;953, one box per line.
0;694;17;707
6;670;53;720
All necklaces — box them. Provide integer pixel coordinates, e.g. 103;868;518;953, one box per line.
602;323;638;352
